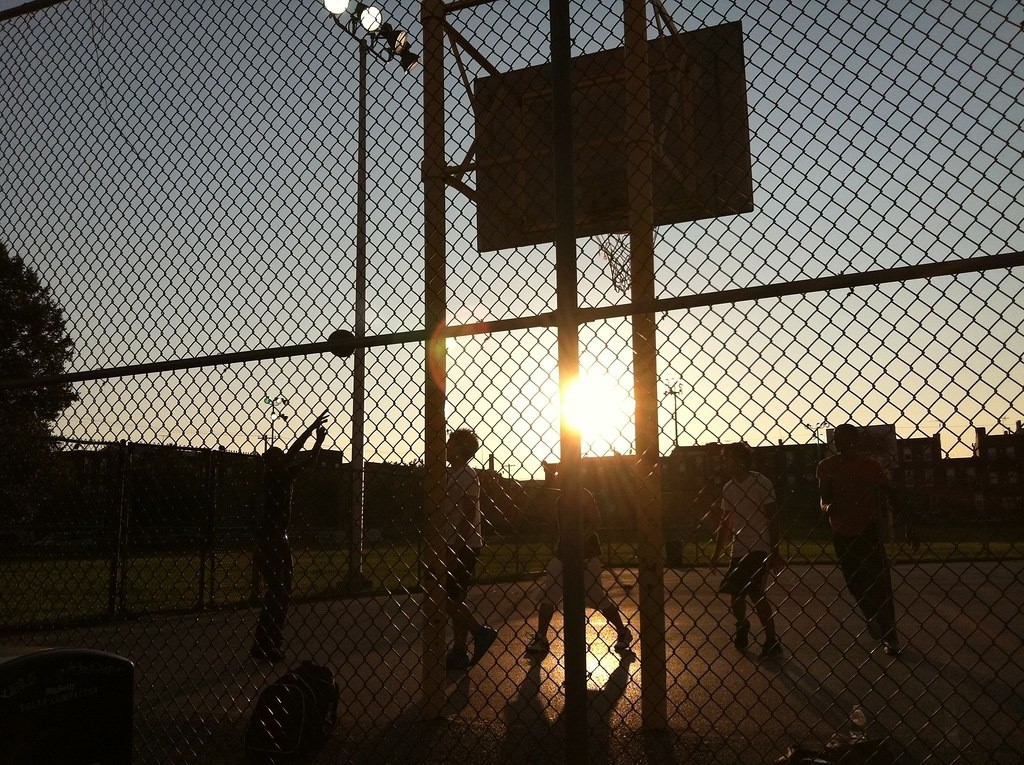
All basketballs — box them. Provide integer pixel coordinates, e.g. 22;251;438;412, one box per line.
328;330;357;358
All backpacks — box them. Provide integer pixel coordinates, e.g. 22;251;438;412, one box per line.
244;662;339;764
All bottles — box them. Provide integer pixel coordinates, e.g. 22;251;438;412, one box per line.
823;731;844;748
850;701;867;743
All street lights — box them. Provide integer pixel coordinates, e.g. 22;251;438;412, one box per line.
805;421;831;463
320;0;421;595
665;380;683;446
265;396;290;448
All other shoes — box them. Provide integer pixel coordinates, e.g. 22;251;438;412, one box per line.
733;619;750;650
866;616;900;655
759;639;782;660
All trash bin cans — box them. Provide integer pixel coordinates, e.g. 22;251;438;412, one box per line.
0;645;137;765
665;539;683;566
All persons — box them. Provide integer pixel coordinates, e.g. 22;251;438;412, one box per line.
714;442;788;659
246;407;331;668
407;429;501;668
521;455;634;656
812;423;919;656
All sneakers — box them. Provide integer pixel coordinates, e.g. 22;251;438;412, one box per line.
446;652;469;669
469;626;496;666
250;645;286;661
526;632;549;652
614;628;633;650
